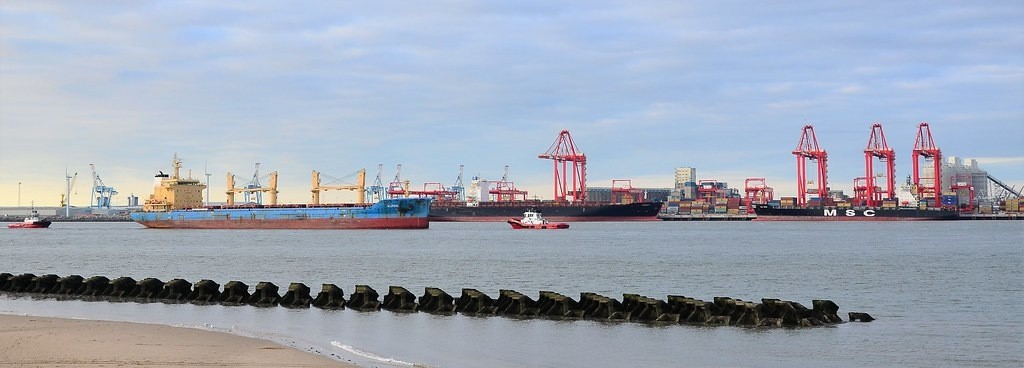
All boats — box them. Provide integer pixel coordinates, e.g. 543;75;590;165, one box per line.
131;154;429;230
507;203;570;230
656;182;757;220
7;201;51;228
749;123;1024;221
429;199;665;222
